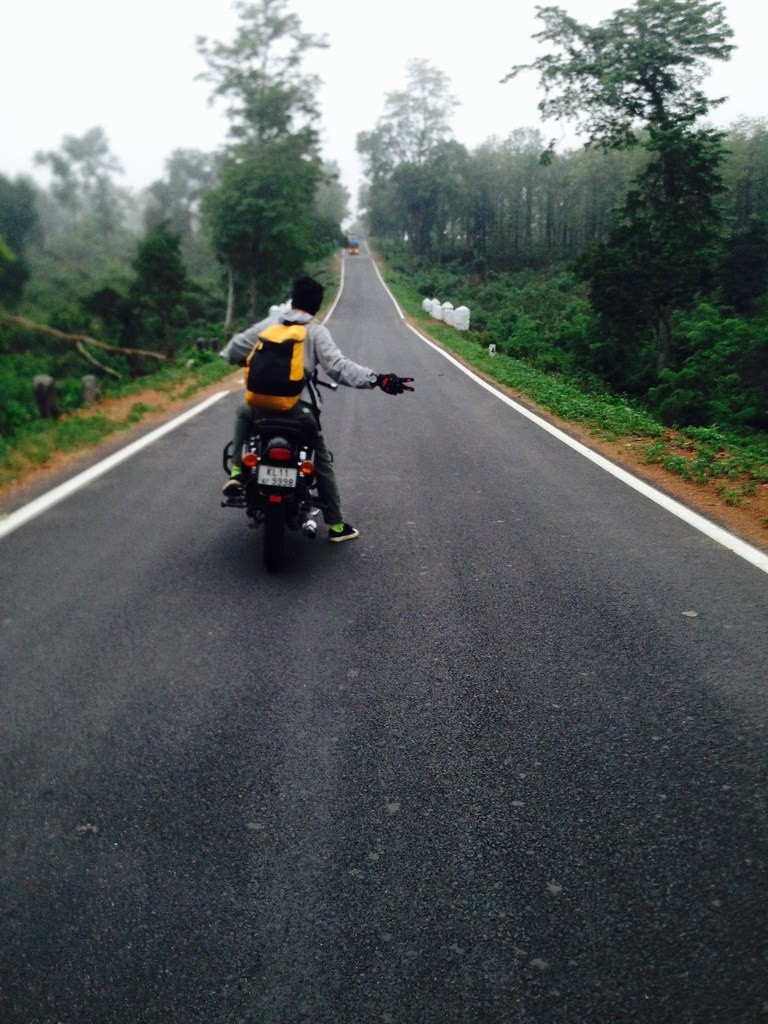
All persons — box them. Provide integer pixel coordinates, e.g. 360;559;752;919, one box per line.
222;276;415;542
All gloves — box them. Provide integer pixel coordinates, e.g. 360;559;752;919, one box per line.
377;372;415;396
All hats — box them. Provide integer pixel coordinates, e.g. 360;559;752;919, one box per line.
291;277;326;314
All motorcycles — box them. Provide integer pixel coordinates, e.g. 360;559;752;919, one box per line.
215;351;339;569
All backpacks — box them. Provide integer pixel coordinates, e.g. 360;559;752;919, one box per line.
244;321;319;410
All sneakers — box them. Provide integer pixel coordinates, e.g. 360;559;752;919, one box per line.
328;523;359;543
222;473;243;496
